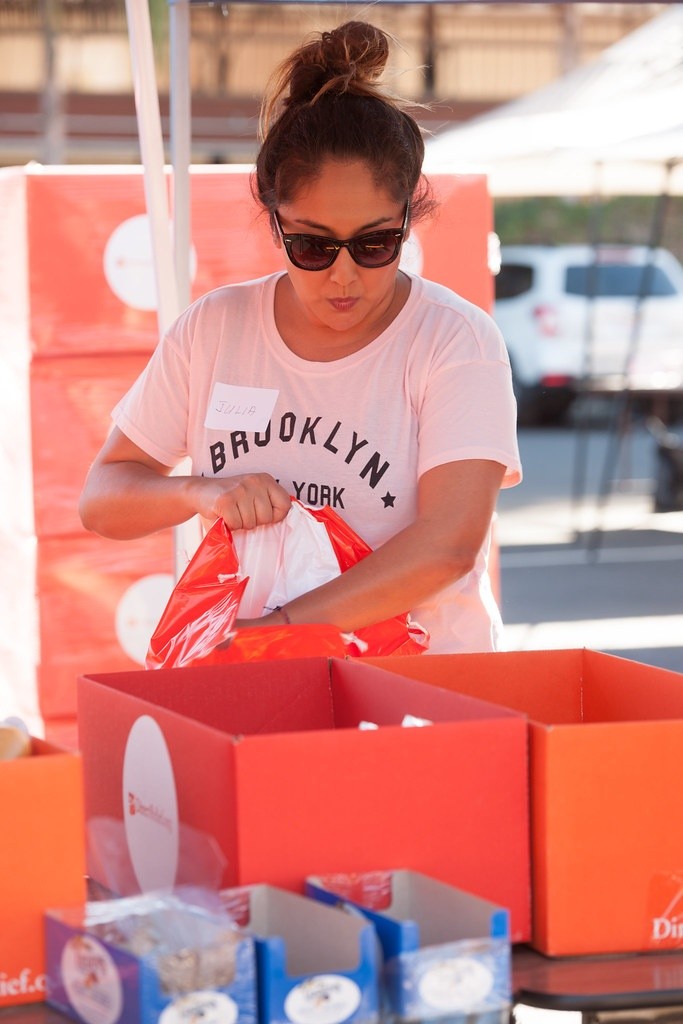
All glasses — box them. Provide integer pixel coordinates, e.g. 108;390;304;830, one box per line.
273;197;409;271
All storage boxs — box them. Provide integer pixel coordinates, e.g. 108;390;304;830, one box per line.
75;653;534;948
42;888;259;1024
347;647;683;959
217;879;383;1024
0;165;510;735
0;724;90;1008
301;867;514;1024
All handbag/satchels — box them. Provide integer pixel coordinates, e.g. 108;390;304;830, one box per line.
146;497;432;670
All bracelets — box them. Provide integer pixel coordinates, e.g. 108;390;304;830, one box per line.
264;606;292;626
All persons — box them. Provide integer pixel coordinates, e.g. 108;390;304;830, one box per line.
79;20;522;656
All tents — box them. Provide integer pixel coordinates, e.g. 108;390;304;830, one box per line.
421;1;683;568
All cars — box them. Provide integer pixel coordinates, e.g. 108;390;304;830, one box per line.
494;242;683;426
